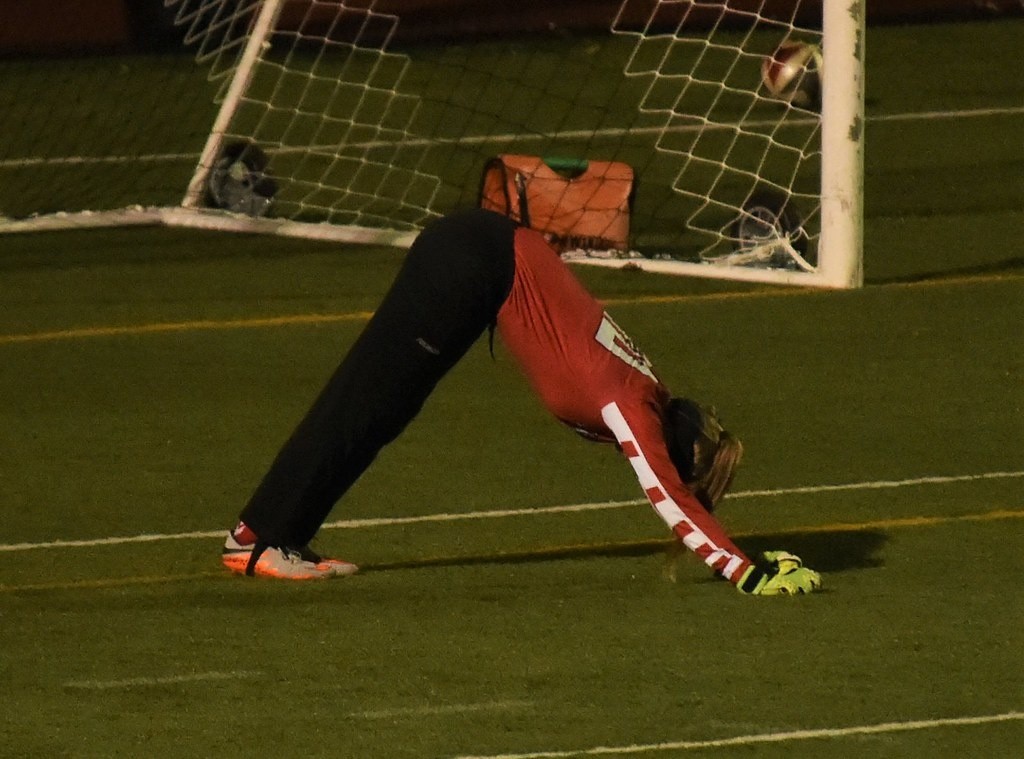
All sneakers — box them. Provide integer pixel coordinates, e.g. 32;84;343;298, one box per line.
223;531;336;580
287;547;358;576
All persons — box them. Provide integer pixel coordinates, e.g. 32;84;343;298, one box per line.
219;205;825;597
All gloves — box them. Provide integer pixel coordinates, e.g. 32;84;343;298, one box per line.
737;566;822;595
754;550;801;575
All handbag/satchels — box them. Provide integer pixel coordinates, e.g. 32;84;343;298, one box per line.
478;154;635;247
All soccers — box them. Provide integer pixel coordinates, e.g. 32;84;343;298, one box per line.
760;41;823;106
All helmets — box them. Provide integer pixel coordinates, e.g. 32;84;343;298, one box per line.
208;141;278;220
